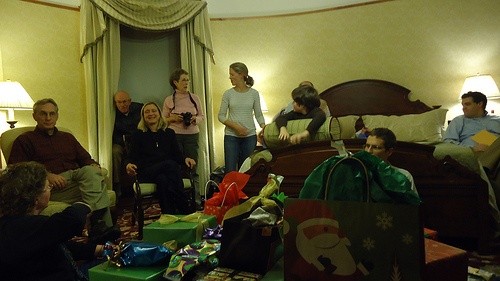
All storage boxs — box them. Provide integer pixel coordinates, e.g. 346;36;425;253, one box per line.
89;195;472;281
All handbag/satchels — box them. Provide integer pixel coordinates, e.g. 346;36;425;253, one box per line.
282;157;427;281
202;180;239;225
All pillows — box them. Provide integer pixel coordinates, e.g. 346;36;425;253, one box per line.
339;113;359;140
362;105;450;144
261;116;337;147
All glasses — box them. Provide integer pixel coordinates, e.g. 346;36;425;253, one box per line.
363;142;385;152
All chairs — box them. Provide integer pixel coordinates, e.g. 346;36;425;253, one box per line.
0;127;114;226
122;128;196;238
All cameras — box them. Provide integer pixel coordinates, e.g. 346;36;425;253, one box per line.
180;112;194;126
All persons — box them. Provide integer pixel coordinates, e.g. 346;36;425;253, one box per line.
123;101;196;216
273;81;330;121
112;91;145;224
0;162;95;281
7;98;117;236
276;86;326;144
365;127;418;194
162;69;203;173
443;92;500;211
218;62;265;176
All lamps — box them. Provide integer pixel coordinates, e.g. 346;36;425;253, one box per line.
0;80;35;130
459;74;500;114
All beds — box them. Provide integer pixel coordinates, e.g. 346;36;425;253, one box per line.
269;79;442;204
